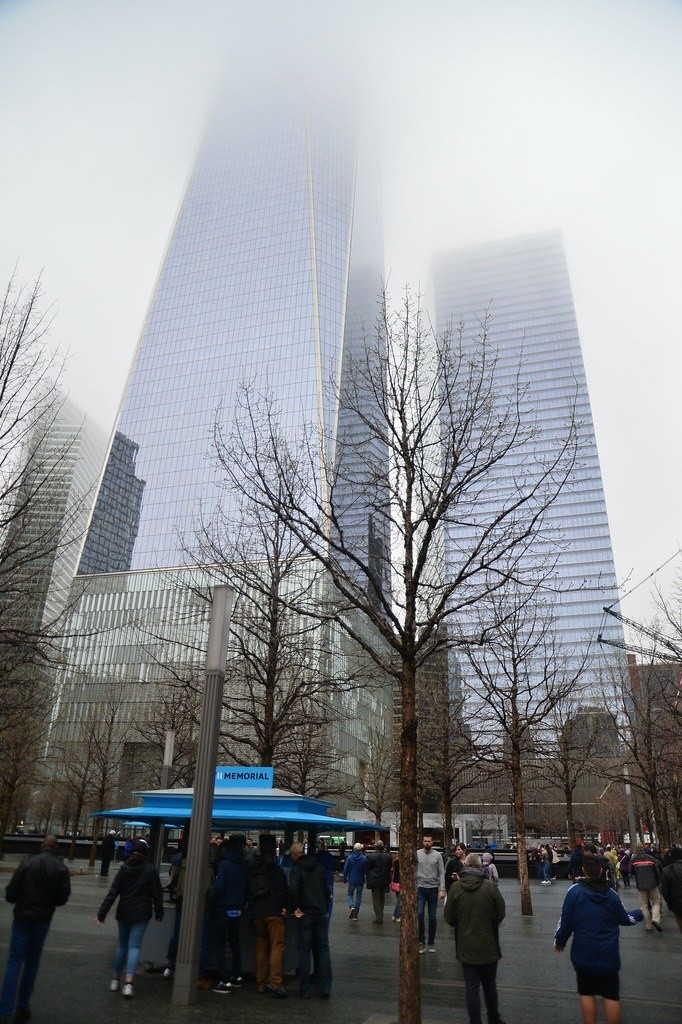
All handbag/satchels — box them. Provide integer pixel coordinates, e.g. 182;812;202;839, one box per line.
390;882;400;892
616;862;621;869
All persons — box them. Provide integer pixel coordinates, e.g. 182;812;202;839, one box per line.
661;847;682;933
342;843;368;920
568;841;671;916
554;854;644;1024
531;844;560;884
96;833;165;996
444;853;506;1024
161;833;335;1000
365;840;392;924
481;853;499;886
0;835;71;1024
484;846;496;867
629;842;663;933
391;857;400;921
416;834;446;954
445;843;469;892
99;830;116;876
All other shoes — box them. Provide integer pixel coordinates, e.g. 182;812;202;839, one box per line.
392;916;396;921
428;944;436;952
353;916;360;920
541;880;551;884
652;921;662;932
122;984;135;997
419;942;426;953
198;967;330;1000
163;968;174;978
395;918;400;922
110;980;120;991
647;929;652;934
349;906;355;919
373;919;383;923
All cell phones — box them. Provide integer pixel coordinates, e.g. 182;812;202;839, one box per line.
452;873;457;876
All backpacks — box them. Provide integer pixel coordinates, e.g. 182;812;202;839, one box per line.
481;861;492;881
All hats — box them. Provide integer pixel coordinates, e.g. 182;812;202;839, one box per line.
133;839;150;853
482;852;493;865
354;843;363;850
284;842;305;855
